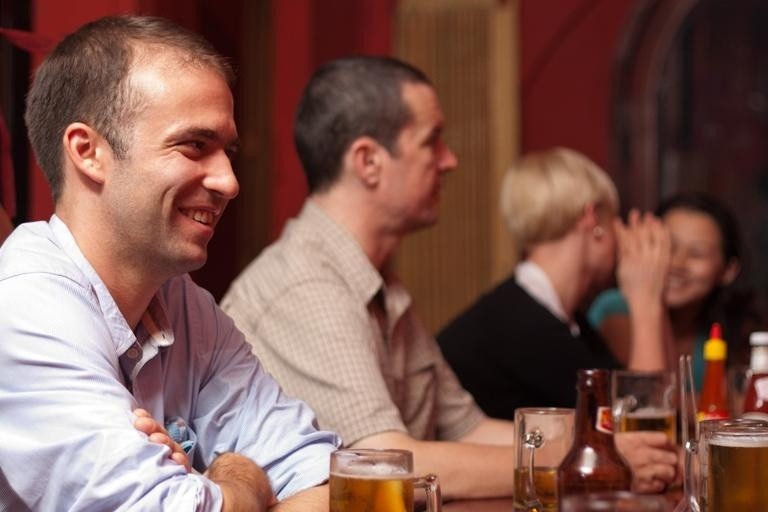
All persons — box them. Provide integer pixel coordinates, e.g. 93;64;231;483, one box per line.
437;148;675;424
590;189;742;417
1;17;348;506
223;51;698;497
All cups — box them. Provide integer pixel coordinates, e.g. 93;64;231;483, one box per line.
513;407;577;507
685;419;768;512
330;450;439;512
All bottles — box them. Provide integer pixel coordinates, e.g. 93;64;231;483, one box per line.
553;369;629;512
737;333;767;417
699;324;731;445
